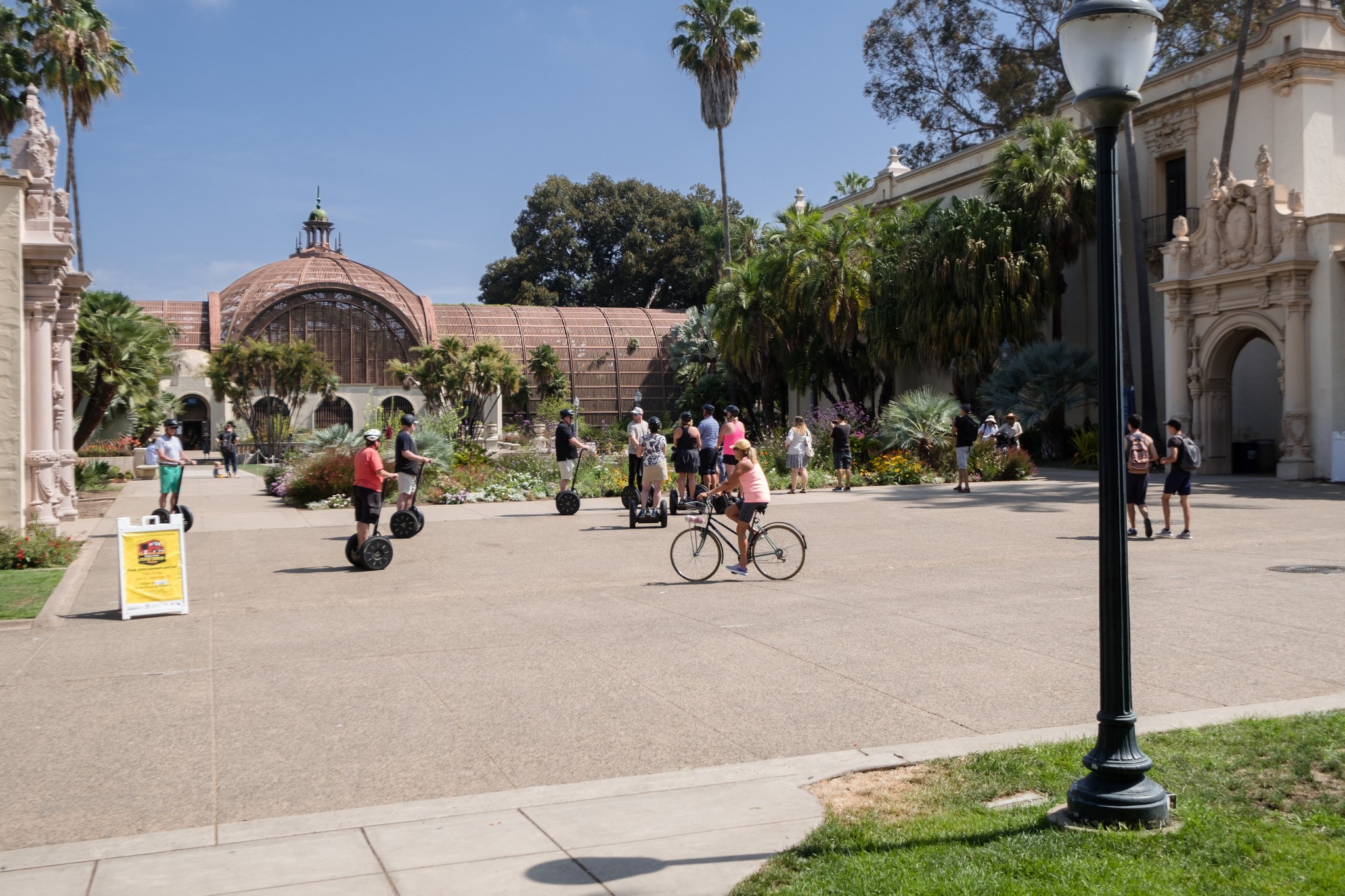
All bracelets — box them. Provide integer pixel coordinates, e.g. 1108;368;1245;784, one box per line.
722;490;727;494
707;491;711;497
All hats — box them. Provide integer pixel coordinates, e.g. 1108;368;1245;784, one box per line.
630;407;643;414
729;438;751;451
203;433;209;436
1162;418;1182;429
958;405;1018;423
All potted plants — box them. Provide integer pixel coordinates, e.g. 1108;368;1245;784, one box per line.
472;421;484;437
486;421;497;434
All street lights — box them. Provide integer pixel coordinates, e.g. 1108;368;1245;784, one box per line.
1050;0;1176;836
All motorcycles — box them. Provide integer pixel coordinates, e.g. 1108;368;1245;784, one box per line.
982;432;1018;455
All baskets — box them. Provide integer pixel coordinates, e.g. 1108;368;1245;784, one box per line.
685;501;711;524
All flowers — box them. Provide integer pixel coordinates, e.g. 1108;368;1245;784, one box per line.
532;414;551;425
501;423;518;432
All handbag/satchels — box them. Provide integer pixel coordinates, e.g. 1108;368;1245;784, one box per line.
805;428;815;457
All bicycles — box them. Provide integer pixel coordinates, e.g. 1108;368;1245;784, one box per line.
670;494;807;581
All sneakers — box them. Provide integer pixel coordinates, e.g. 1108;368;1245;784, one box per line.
1124;519;1191;538
953;486;970;493
725;562;748;575
748;531;765;546
638;500;684;516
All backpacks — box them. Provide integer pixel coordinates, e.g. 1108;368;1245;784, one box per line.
1125;433;1150;474
963;416;978;441
1172;435;1201;471
220;432;234;453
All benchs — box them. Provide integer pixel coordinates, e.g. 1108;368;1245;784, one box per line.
136;464;159;480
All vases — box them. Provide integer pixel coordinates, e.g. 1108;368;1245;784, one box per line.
534;424;546;436
503;431;519;444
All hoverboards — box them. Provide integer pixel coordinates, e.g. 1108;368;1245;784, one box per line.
390;459;431;539
668;446;742;515
344;475;398;571
555;448;588;515
149;461;194;535
621;456;667;528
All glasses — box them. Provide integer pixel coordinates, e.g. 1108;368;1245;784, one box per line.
168;427;177;429
631;413;640;416
1006;417;1014;420
566;416;573;420
733;448;739;451
679;418;681;420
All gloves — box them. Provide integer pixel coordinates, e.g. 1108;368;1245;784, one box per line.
990;434;1014;442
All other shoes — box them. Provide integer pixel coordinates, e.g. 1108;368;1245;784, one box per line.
787;487;851;494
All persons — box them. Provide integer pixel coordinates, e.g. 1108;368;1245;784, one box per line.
696;438;770;576
394;414;433;511
145;424;240;479
784;416;812;494
1124;414;1160;537
830;412;851;492
990;413;1023;453
153;419;197;516
351;428;399;559
627;403;746;517
978;415;998;443
1155;418;1193;539
951;404;973;493
555;409;591;493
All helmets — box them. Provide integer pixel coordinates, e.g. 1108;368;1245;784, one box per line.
164;419;179;428
363;414;419;441
560;409;575;417
648;404;739;429
